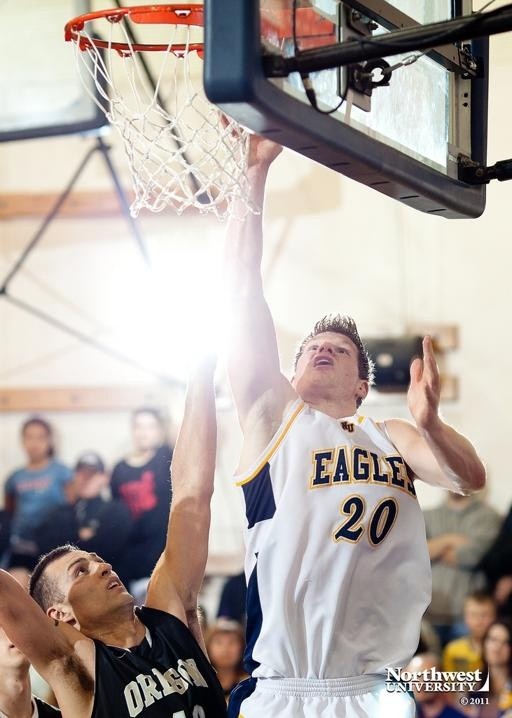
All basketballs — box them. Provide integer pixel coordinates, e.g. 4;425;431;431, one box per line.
257;0;337;50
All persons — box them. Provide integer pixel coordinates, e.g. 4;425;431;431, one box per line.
1;401;509;717
224;105;487;718
0;318;234;718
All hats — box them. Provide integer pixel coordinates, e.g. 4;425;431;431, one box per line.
77;455;103;472
